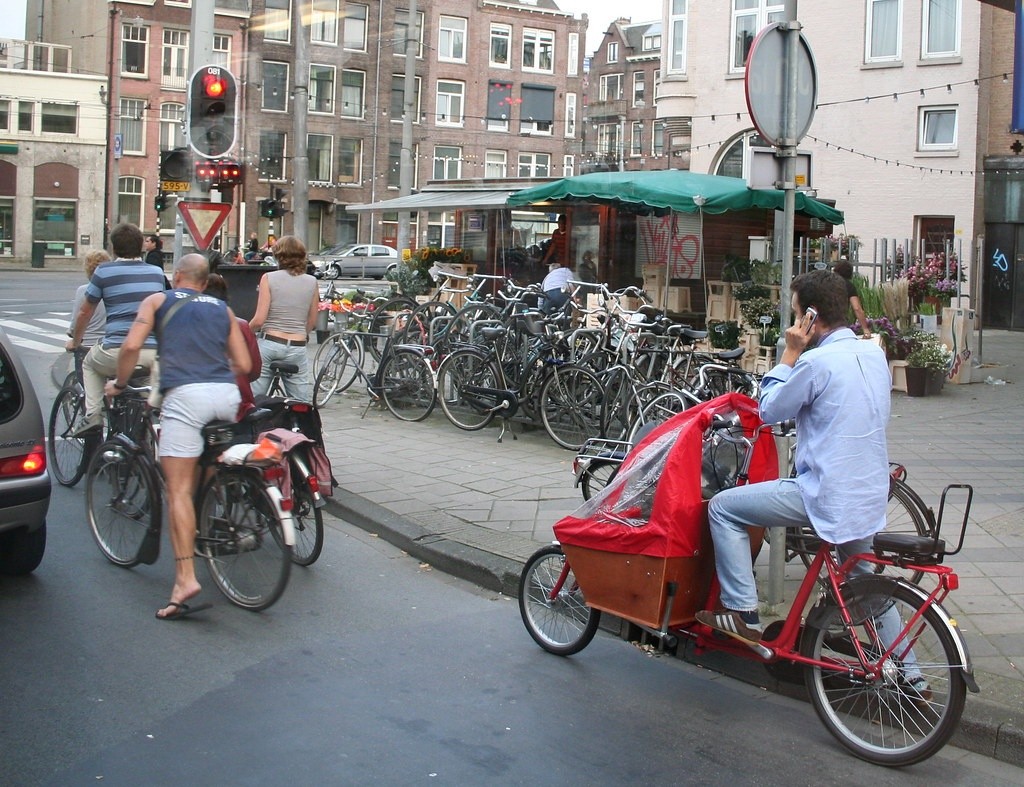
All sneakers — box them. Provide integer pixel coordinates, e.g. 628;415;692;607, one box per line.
899;677;933;706
72;414;104;438
695;610;761;647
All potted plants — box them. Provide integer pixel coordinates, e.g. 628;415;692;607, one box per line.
918;303;938;331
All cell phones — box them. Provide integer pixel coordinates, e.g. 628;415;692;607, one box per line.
800;307;818;334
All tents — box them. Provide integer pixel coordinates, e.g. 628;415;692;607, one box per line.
503;163;842;331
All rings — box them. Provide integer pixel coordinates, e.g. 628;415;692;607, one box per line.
792;325;795;327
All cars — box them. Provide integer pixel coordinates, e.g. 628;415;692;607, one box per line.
0;325;52;577
306;244;404;282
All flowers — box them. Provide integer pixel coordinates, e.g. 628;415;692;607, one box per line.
316;298;374;315
884;244;971;298
812;232;862;252
851;317;954;365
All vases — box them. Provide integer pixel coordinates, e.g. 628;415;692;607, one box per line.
905;366;926;398
888;360;909;392
335;312;349;331
318;310;328;330
928;369;944;394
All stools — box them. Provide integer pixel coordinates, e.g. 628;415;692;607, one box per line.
586;263;691;329
413;264;477;332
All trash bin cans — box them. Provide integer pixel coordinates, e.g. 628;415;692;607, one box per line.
31;240;47;268
219;265;277;319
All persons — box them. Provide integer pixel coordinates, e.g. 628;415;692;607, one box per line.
537;263;578;315
66;225;167;462
828;258;873;339
579;251;598;304
144;234;174;291
250;235;320;405
201;271;262;424
244;233;258;261
696;269;936;708
541;212;567;268
104;254;253;617
70;250;108;345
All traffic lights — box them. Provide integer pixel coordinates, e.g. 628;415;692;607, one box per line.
155;196;165;211
264;199;277;219
275;187;291;218
160;151;193;182
186;65;240;159
195;162;245;185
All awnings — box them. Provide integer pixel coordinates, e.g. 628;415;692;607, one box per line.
345;186;520;216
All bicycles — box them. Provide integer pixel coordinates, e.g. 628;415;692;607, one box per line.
312;269;936;600
48;329;327;568
85;380;297;612
518;417;980;768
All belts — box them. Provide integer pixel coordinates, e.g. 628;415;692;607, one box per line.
260;332;306;346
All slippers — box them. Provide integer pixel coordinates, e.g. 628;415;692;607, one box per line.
155;602;212;620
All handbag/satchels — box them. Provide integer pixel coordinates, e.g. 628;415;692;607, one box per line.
147;356;164;409
257;427;338;510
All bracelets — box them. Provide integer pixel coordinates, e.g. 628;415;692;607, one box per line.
113;379;128;390
